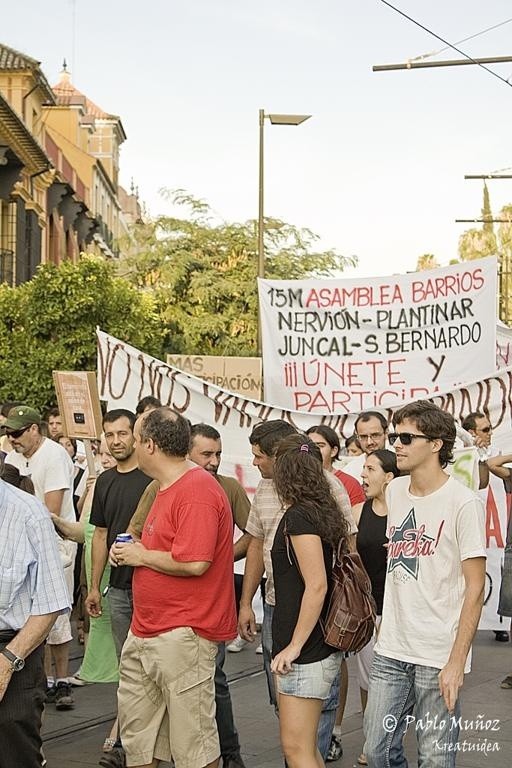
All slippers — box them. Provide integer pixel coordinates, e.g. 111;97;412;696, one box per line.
501;675;512;689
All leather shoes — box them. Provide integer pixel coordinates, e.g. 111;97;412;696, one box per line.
495;632;509;643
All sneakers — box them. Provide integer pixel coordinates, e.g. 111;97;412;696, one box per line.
255;643;264;654
45;685;56;703
327;735;343;761
69;665;94;686
55;684;76;710
227;633;249;653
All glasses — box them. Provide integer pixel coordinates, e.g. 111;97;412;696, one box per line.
357;432;385;439
474;424;493;433
5;426;32;439
387;433;433;445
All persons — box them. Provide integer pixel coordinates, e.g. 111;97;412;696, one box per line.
83;409;154;664
125;424;252;768
1;404;18;455
230;574;268;654
485;454;512;689
345;435;362;456
238;419;359;768
0;406;77;710
46;411;63;436
461;413;508;641
341;411;396;484
0;476;72;768
77;433;116;684
110;407;237;767
303;425;366;528
360;400;487;768
51;432;85;606
136;395;161;416
268;432;372;768
356;449;404;765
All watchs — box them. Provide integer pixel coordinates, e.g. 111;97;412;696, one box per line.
2;648;25;672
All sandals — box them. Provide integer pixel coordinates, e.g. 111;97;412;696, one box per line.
101;738;115;754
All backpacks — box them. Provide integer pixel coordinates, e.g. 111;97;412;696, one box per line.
281;510;375;653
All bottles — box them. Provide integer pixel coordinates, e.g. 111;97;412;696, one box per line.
115;534;134;545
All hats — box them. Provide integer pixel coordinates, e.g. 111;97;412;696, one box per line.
0;405;41;430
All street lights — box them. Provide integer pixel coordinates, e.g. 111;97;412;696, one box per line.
254;108;312;356
